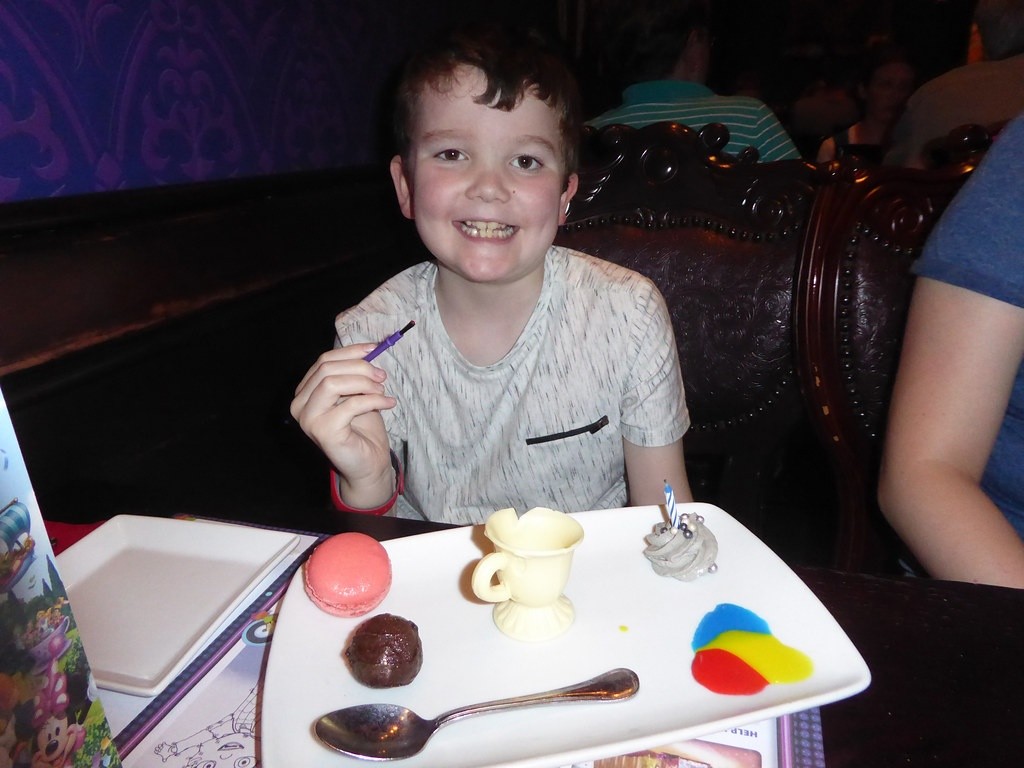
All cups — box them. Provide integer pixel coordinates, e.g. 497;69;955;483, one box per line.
471;505;584;640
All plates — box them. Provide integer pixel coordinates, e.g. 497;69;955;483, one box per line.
260;501;872;768
54;514;302;698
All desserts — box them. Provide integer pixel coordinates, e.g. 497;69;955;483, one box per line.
304;532;393;618
345;613;423;689
644;513;717;582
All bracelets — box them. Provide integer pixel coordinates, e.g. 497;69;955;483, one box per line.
328;447;404;515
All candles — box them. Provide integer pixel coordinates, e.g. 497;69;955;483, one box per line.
663;477;681;529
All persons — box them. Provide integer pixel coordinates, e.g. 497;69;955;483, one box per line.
877;112;1024;590
290;37;695;527
579;0;801;165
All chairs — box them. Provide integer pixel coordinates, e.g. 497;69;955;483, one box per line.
793;119;1013;574
553;122;815;541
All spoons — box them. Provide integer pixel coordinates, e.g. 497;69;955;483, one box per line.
315;668;640;761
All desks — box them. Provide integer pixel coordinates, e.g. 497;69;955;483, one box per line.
41;517;1023;768
